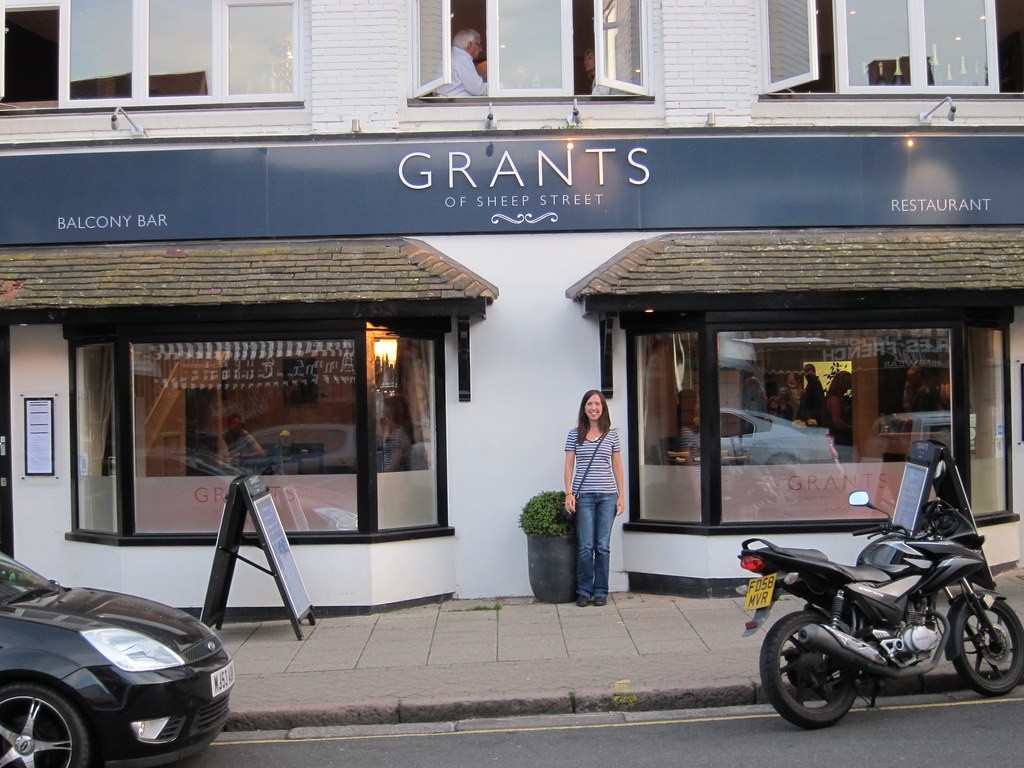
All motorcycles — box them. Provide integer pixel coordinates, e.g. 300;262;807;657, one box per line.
736;489;1024;730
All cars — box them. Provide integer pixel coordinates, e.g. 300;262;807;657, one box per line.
1;550;236;768
700;407;839;465
221;422;356;473
139;446;243;477
860;413;951;463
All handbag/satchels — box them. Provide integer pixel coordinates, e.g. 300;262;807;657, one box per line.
560;501;579;523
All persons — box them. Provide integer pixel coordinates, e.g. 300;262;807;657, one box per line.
222;413;265;469
742;359;945;461
563;390;624;607
431;28;487;98
677;389;700;513
380;394;415;472
583;49;594;95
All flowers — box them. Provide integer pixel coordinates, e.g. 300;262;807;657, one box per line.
791;418;818;431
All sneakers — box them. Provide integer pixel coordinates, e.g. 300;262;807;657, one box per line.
577;595;589;607
593;596;607;606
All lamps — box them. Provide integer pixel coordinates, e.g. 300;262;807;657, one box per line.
918;96;956;125
109;107;145;136
351;119;361;133
703;110;716;127
485;102;498;129
566;99;583;128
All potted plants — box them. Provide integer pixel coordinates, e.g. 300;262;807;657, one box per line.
517;489;580;604
